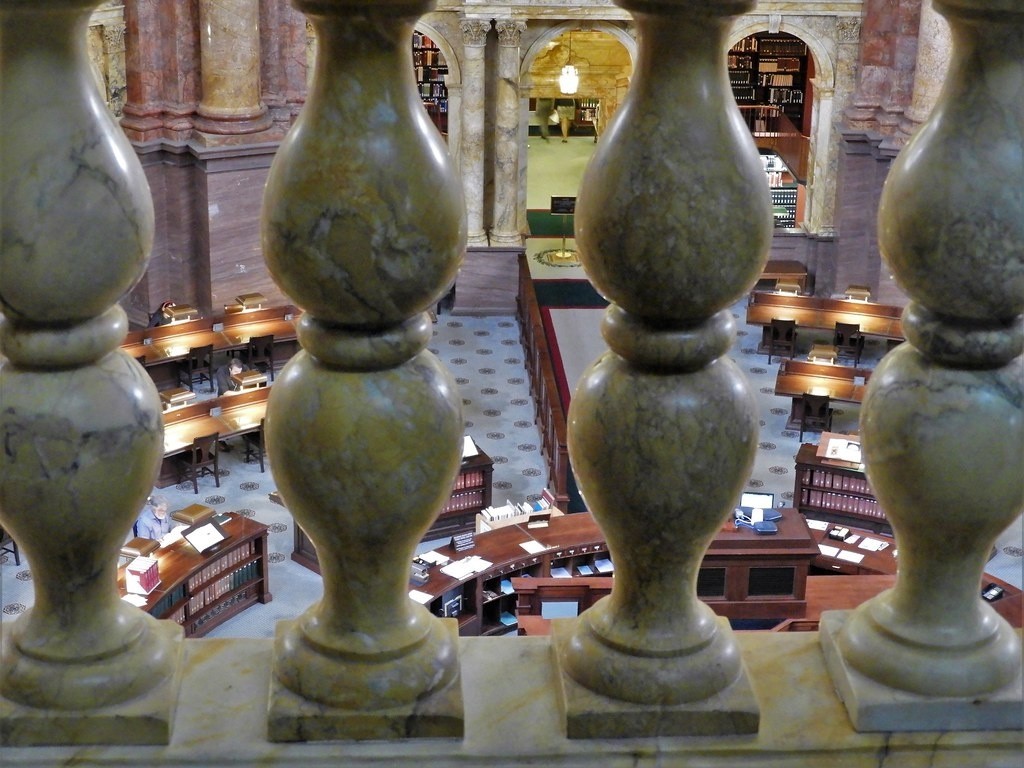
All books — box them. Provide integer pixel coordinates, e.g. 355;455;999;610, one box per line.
440;469;484;515
126;555;160;593
767;189;797;228
412;30;449;116
580;98;600;122
727;38;808;111
480;496;550;522
800;468;890;521
148;541;261;638
212;513;233;525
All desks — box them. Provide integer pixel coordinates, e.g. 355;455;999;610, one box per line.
746;291;906;356
0;386;273;550
759;260;807;295
775;358;873;431
118;304;438;368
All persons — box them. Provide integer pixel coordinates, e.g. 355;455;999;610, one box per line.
217;358;250;397
557;99;576;143
147;301;177;328
535;98;555;140
137;495;176;541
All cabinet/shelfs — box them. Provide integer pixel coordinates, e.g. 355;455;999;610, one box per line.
728;32;809;133
412;30;449;133
408;512;1024;636
528;97;601;138
268;437;496;576
793;443;895;539
118;511;273;639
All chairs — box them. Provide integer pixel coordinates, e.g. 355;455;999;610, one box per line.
240;335;275;382
768;318;798;364
132;520;171;540
177;343;215;392
833;320;863;369
243;418;266;473
178;432;220;493
800;392;833;442
0;523;20;566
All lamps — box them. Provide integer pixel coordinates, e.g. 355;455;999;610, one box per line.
164;304;198;323
171;503;217;525
808;344;839;365
845;285;871;302
158;388;196;409
560;31;579;94
235;292;268;311
775;278;801;295
119;537;161;559
231;371;268;390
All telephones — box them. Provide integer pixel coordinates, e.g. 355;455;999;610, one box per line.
982;583;1005;601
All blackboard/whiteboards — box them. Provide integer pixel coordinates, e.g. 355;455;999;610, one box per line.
550;196;576;215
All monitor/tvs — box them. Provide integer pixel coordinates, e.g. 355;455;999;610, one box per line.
740;492;774;509
